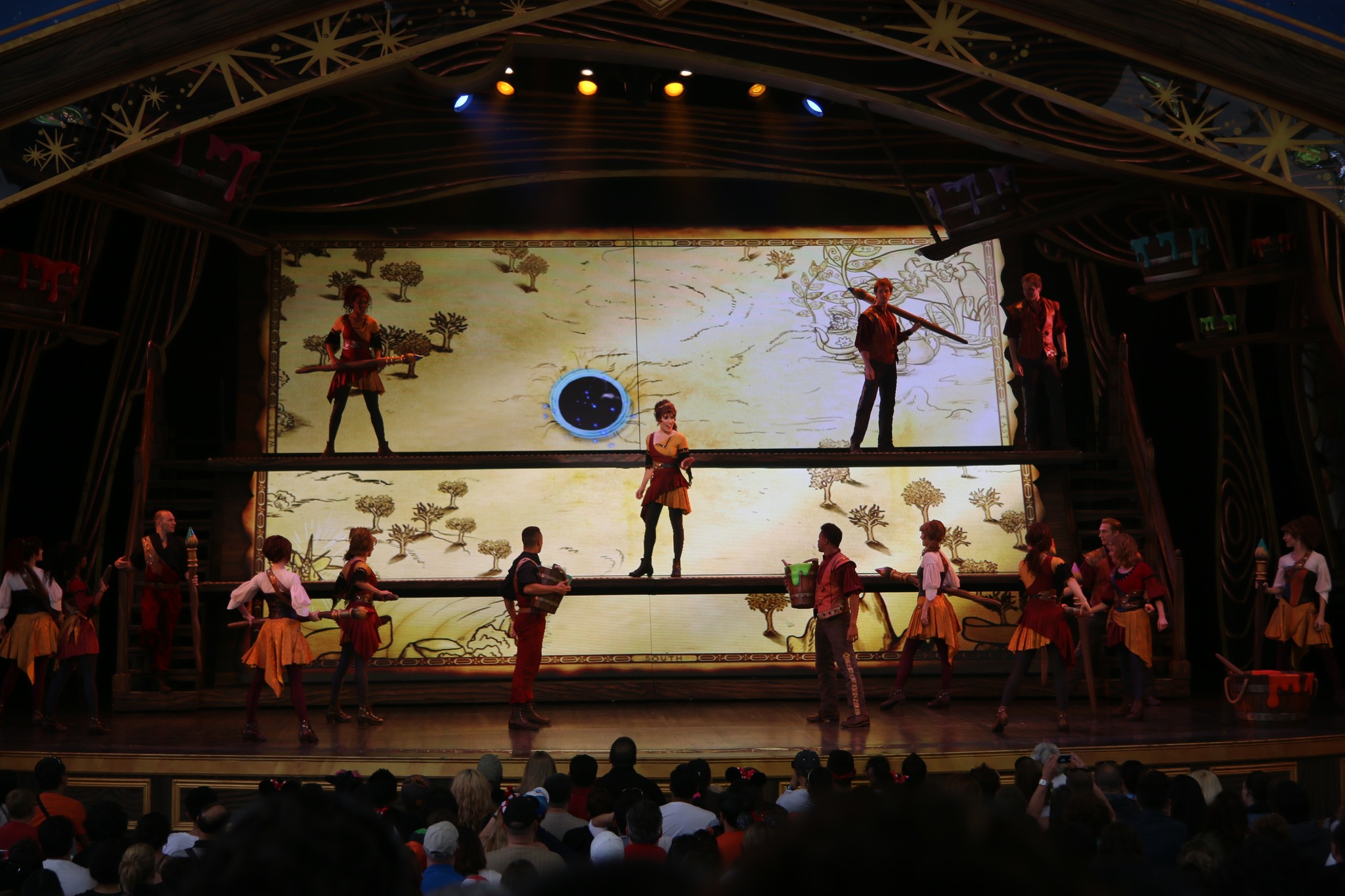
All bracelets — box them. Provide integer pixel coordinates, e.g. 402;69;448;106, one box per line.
906;330;912;336
1061;351;1068;356
99;590;104;594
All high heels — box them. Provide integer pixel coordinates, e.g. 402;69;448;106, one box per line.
298;727;319;743
242;723;267;742
325;706;352;723
928;693;952;710
1126;706;1144;720
993;709;1009;734
1112;701;1131;717
880;694;909;711
1058;710;1069;730
356;707;384;727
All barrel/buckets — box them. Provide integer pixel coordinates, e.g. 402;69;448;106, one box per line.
1225;670;1318;727
782;559;819;609
530;564;572;614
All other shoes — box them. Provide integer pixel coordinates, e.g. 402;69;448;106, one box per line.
1146;695;1162;705
850;444;862;455
806;710;840;723
89;721;113;735
840;715;871;728
877;444;899;452
33;711;47;723
48;722;68;732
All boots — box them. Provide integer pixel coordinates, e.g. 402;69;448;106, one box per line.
377;441;393;456
508;704;540;730
522;705;552;725
672;560;683;577
320;441;335;457
629;558;653;577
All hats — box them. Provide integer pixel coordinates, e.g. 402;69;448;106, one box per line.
793;750;819;771
503;795;541;830
423;821;458;859
476;753;503;792
524;787;550;817
590;831;624;867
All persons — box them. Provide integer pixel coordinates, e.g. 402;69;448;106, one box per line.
227;535;322;745
1060;518;1168;721
325;528;393;726
501;526;571;730
990;522;1092;734
64;545;111;734
850;278;926;454
318;284;399;458
629;399;694;578
879;520;963;710
784;523;872;728
1255;516;1333;672
109;510;199;692
0;735;1345;896
0;535;67;732
1003;272;1073;449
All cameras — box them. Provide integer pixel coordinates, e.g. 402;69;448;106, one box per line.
1058;754;1071;763
